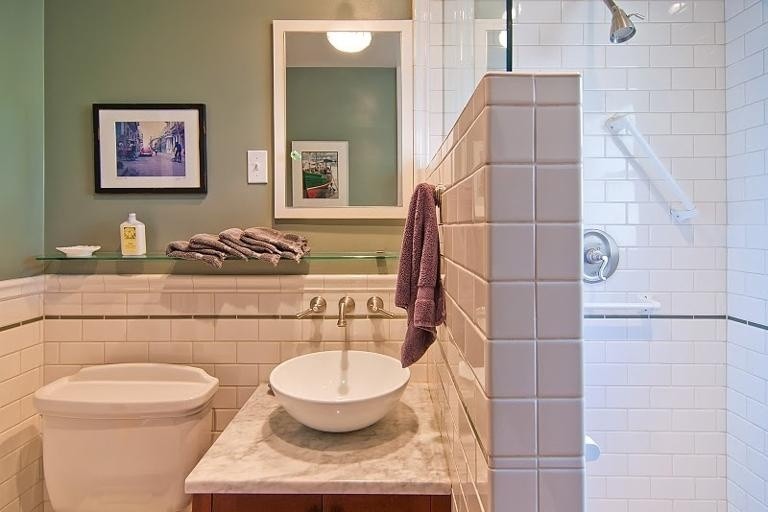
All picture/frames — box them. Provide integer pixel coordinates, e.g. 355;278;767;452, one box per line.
89;97;210;199
290;138;349;205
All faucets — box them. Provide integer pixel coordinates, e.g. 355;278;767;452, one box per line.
336;297;355;327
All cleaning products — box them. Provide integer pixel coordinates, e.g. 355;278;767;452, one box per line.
119;213;146;255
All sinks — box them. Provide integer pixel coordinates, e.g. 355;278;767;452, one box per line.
269;350;410;433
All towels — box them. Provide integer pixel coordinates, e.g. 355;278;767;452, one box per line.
389;182;449;366
162;221;308;271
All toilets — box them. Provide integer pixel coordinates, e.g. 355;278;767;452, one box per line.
35;362;220;512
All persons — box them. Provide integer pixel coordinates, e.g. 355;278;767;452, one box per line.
174;141;181;162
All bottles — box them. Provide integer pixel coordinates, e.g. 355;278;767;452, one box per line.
117;211;147;258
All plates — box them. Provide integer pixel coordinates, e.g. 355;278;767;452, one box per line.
56;244;100;258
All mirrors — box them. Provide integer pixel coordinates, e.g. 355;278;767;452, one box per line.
271;18;412;223
